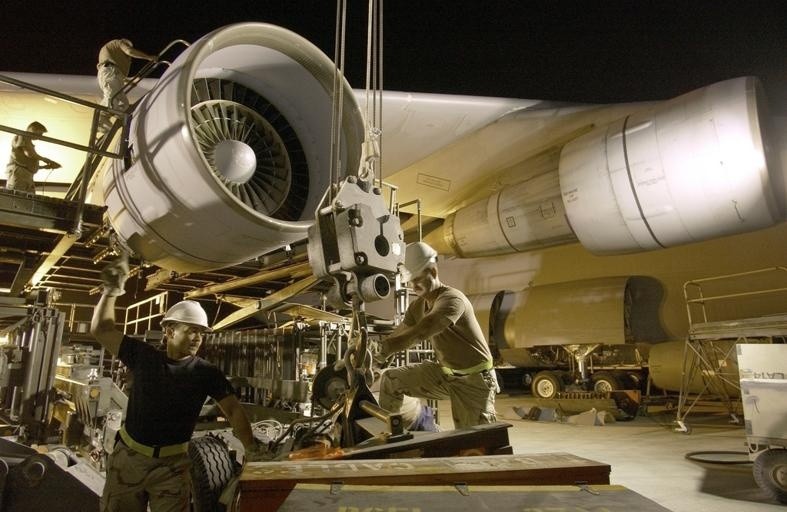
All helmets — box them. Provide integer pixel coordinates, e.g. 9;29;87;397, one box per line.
398;242;437;284
160;300;212;330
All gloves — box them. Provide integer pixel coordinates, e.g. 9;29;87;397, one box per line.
102;250;129;296
348;330;381;357
246;445;274;461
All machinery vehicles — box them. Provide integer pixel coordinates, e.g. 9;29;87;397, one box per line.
736;342;786;508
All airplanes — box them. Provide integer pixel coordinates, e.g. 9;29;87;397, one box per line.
1;23;786;404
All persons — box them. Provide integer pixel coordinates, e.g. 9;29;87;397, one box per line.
96;38;160;133
4;121;62;194
377;242;498;431
90;261;253;512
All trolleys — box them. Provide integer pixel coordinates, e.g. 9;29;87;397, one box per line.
675;267;785;435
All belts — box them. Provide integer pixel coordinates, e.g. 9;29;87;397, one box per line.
441;359;493;375
119;423;190;459
